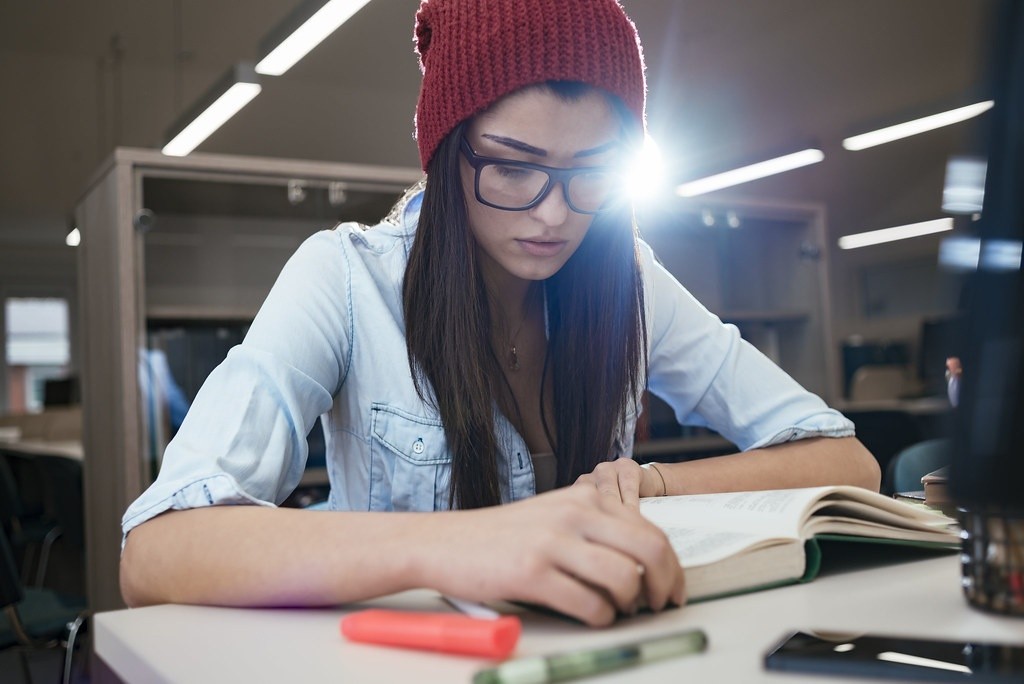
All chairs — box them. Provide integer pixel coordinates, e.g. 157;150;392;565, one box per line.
0;530;92;684
9;456;85;585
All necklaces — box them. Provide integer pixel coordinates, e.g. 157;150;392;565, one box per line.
490;304;531;372
488;335;557;462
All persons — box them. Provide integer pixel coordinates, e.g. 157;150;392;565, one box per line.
120;0;883;627
892;355;962;491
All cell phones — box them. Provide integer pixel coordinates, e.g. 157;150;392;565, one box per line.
766;630;1024;684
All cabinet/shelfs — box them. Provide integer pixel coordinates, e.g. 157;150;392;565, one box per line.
77;147;837;617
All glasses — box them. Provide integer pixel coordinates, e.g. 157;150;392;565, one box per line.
456;128;649;216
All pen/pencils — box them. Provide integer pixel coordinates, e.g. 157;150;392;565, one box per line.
469;629;708;684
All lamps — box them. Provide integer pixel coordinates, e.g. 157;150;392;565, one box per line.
65;0;376;247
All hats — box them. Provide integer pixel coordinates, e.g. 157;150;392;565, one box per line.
410;1;648;175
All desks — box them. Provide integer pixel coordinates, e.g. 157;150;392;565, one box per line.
5;440;82;463
90;539;1024;684
844;395;952;416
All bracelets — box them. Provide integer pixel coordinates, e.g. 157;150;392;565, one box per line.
650;462;678;496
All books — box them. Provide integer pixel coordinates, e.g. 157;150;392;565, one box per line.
438;484;964;620
